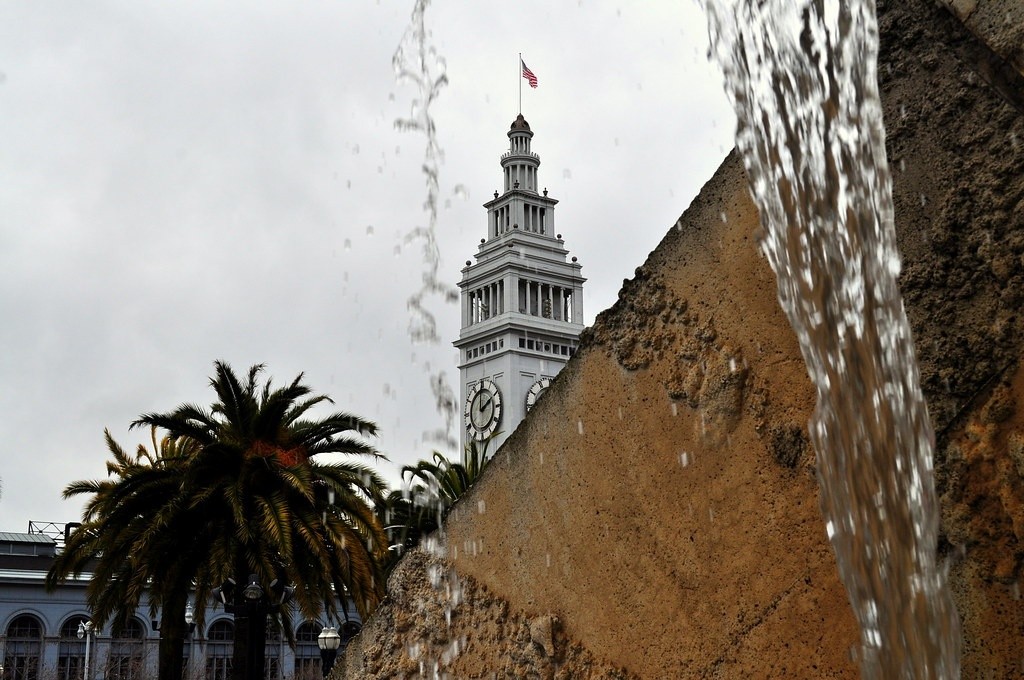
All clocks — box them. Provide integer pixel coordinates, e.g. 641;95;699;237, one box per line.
464;380;504;440
526;377;554;413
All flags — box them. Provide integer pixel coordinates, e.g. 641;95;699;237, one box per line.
522;60;538;90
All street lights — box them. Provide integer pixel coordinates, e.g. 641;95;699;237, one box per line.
77;620;101;680
318;627;340;677
